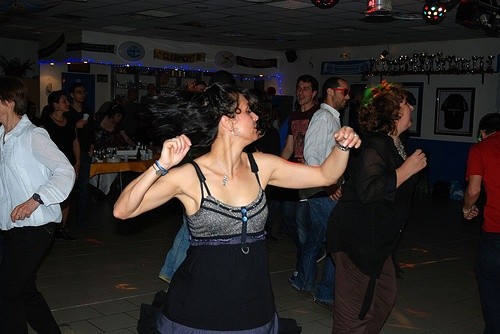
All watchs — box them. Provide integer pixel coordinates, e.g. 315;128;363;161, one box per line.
34;193;44;205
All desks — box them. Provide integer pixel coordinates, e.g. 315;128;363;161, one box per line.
89;151;176;193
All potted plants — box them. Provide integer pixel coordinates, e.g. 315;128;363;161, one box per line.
0;55;33;78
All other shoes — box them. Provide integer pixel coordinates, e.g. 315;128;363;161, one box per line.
289;271;298;281
315;296;334;312
316;251;327;263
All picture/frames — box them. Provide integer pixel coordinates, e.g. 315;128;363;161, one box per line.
349;84;366;133
396;83;424;138
434;87;476;137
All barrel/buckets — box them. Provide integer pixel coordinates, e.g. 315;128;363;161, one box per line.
106;158;121;163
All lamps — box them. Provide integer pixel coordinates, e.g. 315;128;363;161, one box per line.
311;0;500;38
48;82;52;91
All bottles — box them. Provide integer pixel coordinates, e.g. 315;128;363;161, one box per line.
115;79;135;89
92;141;153;164
158;68;185;78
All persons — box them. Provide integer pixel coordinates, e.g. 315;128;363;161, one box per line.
112;82;361;334
281;74;320;281
464;113;500;334
326;83;427;334
40;71;280;241
293;78;351;305
0;75;75;334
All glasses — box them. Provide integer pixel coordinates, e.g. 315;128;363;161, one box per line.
77;91;85;94
333;88;349;96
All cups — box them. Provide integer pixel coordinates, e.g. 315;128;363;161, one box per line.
83;114;88;120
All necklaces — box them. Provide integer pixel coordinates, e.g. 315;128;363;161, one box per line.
213;155;240;185
51;116;65;122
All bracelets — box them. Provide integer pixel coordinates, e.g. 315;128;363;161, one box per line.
152;165;162;176
155;161;168;175
336;141;350;151
462;207;473;213
